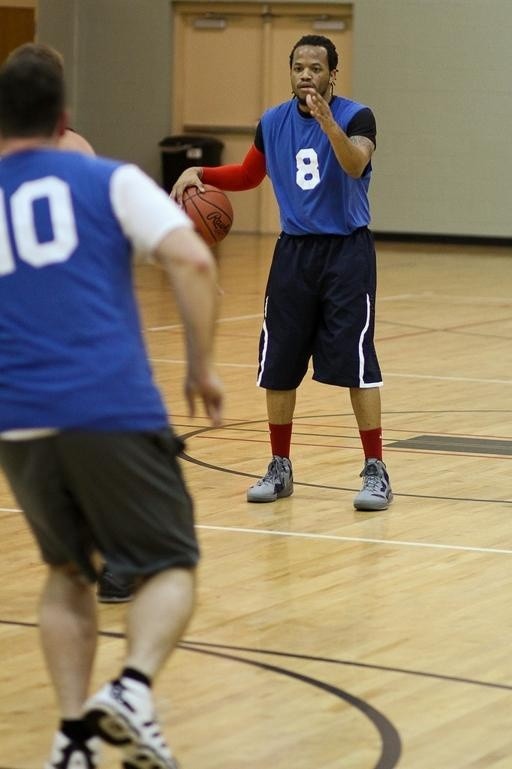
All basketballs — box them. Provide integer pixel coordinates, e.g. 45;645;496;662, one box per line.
175;185;234;246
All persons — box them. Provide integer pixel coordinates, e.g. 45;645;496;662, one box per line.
56;128;99;158
0;40;228;769
164;33;395;510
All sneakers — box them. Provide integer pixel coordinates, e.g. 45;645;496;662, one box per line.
247;455;294;503
353;457;394;511
96;562;138;603
42;728;103;769
79;674;180;769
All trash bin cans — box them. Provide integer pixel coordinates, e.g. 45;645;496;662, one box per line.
157;133;224;195
